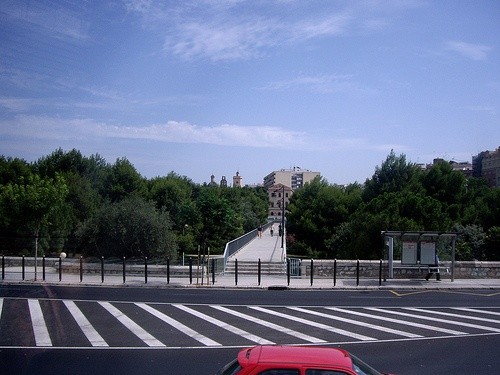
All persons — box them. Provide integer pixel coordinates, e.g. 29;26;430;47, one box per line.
270;224;274;236
278;223;282;236
424;249;442;282
258;224;263;239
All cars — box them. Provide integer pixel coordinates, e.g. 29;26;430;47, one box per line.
218;345;382;375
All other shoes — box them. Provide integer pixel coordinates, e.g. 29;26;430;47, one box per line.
425;277;429;281
436;280;442;281
